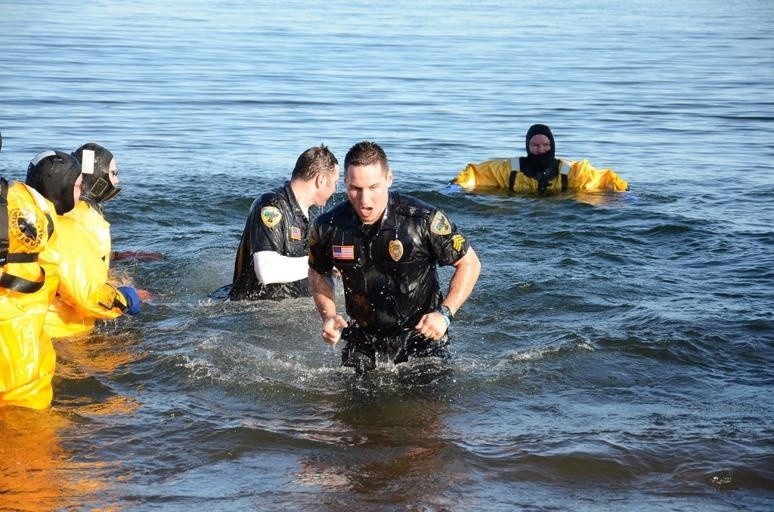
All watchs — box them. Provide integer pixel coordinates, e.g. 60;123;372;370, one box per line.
437;303;455;323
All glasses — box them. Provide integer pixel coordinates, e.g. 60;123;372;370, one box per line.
104;169;120;176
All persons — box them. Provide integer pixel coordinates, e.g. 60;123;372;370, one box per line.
229;141;340;301
24;149;150;338
310;139;482;375
72;140;125;270
443;120;628;197
1;179;64;412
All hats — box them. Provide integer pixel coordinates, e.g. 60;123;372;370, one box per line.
525;124;555;164
26;143;122;216
